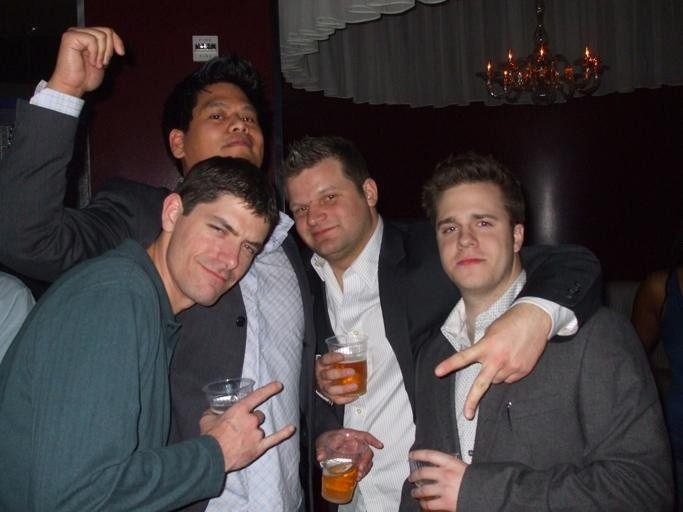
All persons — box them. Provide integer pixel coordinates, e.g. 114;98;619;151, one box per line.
403;153;673;512
270;132;604;512
630;261;683;511
1;154;297;511
0;24;325;511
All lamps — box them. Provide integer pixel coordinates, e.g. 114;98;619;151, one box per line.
484;0;605;106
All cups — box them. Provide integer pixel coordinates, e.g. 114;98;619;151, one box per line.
202;377;256;416
405;452;460;512
324;335;370;397
320;433;369;503
